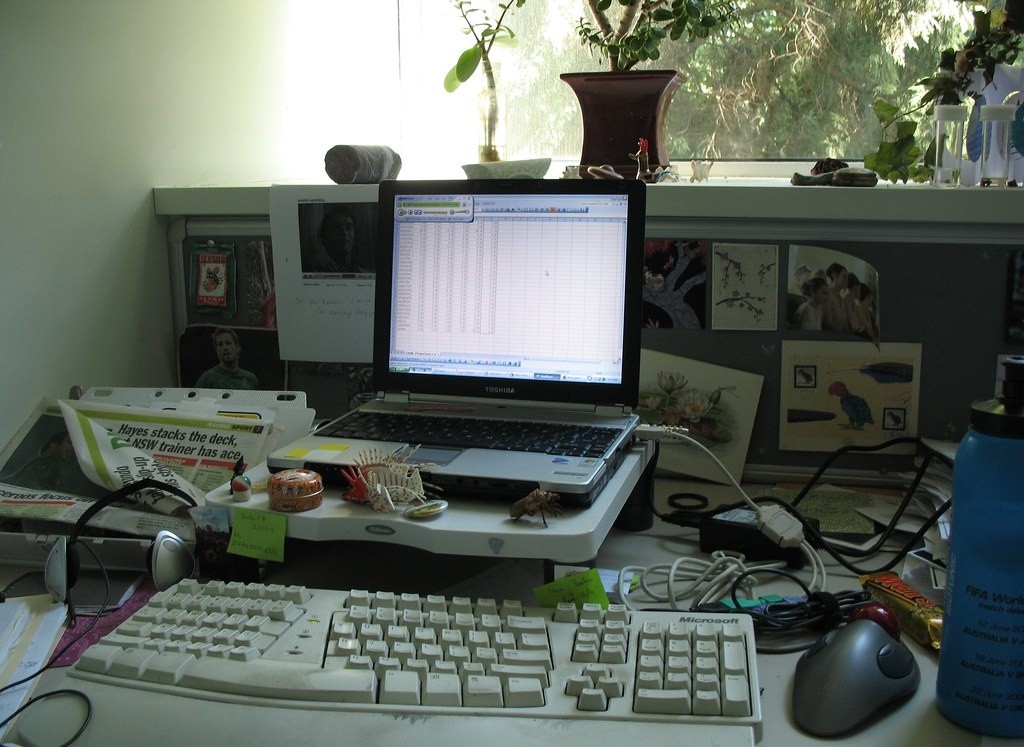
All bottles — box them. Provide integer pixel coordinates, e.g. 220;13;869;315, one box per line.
934;354;1024;739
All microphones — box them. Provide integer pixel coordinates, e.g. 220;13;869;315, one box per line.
56;590;86;629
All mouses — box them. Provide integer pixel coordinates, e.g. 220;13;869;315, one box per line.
789;616;921;740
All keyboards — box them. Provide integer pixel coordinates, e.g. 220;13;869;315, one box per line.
14;573;764;746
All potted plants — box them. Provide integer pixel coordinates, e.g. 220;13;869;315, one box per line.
443;0;551;181
559;0;741;182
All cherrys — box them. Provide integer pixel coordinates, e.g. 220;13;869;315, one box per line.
845;602;902;642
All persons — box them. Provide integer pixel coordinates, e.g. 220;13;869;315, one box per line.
792;264;879;336
195;329;261;391
313;208;374;272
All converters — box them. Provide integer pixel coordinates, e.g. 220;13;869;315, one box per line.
696;503;825;569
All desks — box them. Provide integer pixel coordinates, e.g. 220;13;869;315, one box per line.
0;484;1024;747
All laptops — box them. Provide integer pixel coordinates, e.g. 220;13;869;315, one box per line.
266;180;647;504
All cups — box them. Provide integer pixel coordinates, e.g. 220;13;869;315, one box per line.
979;105;1016;188
934;105;967;186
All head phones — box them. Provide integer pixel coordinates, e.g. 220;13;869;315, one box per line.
46;478;202;605
